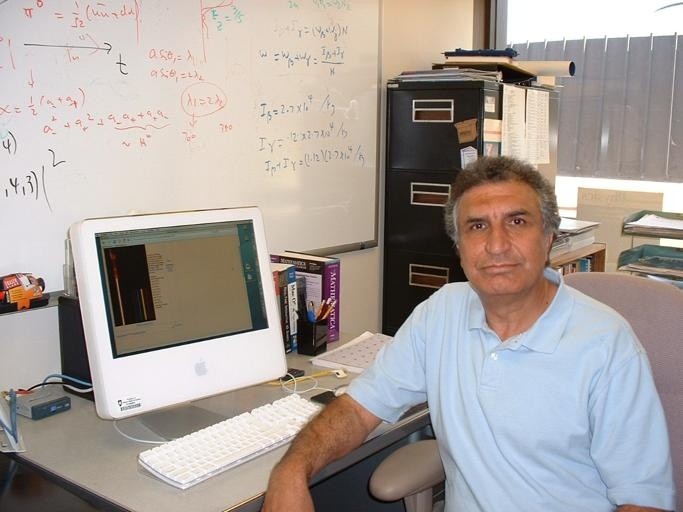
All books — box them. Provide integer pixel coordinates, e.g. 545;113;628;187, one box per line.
546;202;683;279
269;244;339;361
393;50;540;86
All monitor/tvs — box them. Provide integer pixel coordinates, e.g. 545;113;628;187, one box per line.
69;207;287;420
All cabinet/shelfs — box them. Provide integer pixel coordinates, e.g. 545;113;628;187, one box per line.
615;210;681;287
380;83;605;362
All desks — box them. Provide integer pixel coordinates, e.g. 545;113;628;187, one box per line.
0;348;433;512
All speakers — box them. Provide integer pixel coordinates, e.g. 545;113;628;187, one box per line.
58;294;96;402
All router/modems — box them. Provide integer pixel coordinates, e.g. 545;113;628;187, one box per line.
15;384;71;421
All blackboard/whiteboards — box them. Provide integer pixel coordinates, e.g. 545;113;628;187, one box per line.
1;0;385;312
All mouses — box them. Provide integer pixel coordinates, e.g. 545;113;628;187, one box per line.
334;385;347;397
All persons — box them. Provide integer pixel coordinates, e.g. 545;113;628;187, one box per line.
258;158;683;507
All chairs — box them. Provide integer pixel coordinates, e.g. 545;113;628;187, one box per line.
367;271;682;511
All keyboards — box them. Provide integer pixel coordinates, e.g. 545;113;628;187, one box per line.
137;392;325;491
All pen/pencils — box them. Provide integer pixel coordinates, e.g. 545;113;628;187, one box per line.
297;299;339;323
